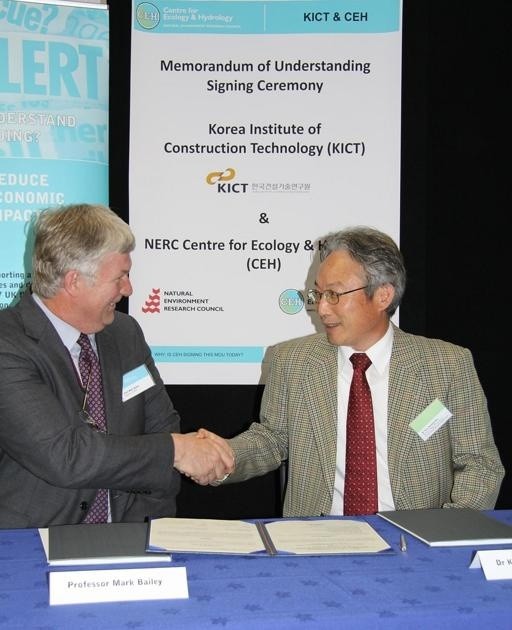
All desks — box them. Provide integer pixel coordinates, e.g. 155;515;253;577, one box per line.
1;509;512;630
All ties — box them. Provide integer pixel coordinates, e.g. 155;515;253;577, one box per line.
77;333;108;524
344;354;378;515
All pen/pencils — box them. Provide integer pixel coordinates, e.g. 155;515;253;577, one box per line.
400;534;407;552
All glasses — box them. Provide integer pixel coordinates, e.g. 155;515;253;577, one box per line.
308;286;368;306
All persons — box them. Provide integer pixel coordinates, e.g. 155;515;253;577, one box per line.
178;228;505;515
1;202;235;523
